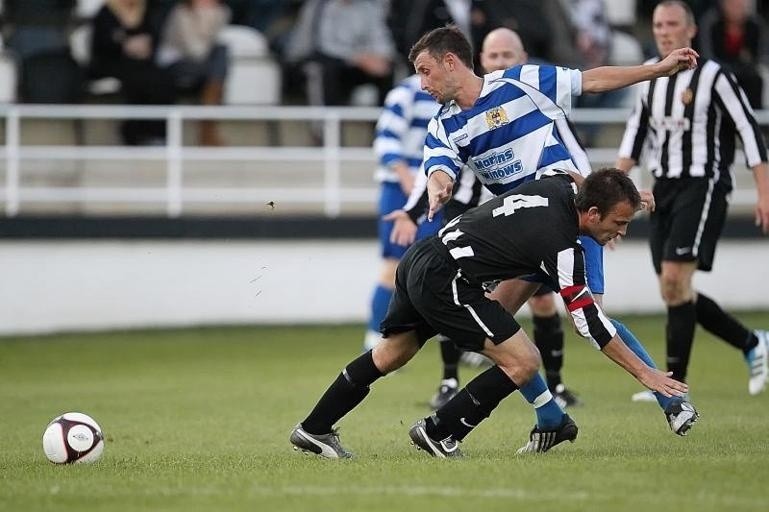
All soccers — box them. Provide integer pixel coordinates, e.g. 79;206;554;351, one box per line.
43;412;103;464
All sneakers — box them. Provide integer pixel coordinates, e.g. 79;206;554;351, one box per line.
289;423;352;458
631;387;699;435
410;376;577;460
745;329;769;395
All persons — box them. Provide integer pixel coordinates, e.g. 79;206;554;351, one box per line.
605;1;769;404
427;27;584;408
288;167;688;461
0;0;769;147
363;73;495;366
407;25;701;454
